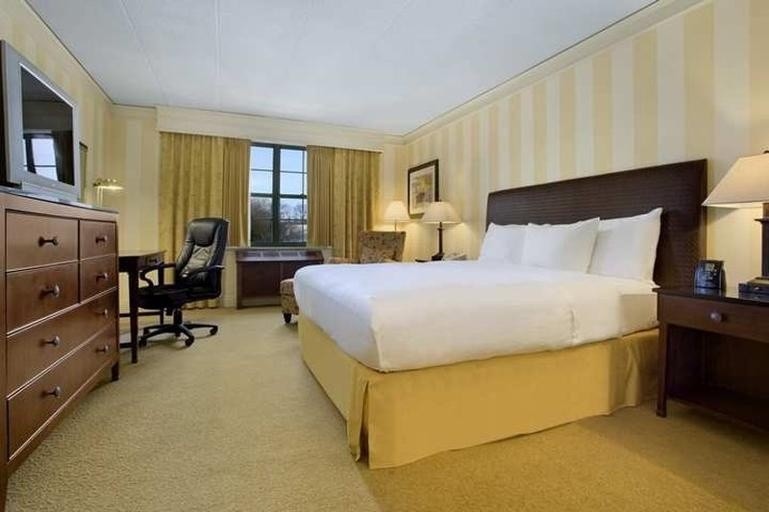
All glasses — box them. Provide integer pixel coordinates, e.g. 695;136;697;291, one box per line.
380;200;410;233
421;202;460;261
90;175;124;205
701;155;768;292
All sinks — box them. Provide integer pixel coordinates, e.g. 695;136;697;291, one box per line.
279;230;406;323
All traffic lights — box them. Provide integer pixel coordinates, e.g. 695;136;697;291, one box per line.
591;207;664;277
475;222;524;267
521;217;602;272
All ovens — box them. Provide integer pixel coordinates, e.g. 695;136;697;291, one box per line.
295;160;707;469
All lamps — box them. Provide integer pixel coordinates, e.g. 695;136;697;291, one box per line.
137;216;229;347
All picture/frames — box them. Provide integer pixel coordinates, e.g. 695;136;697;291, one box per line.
118;249;167;363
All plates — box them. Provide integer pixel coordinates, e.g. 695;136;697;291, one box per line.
0;191;120;512
652;283;767;434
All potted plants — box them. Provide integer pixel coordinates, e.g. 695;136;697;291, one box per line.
407;158;439;216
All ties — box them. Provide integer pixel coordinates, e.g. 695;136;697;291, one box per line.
0;39;80;202
